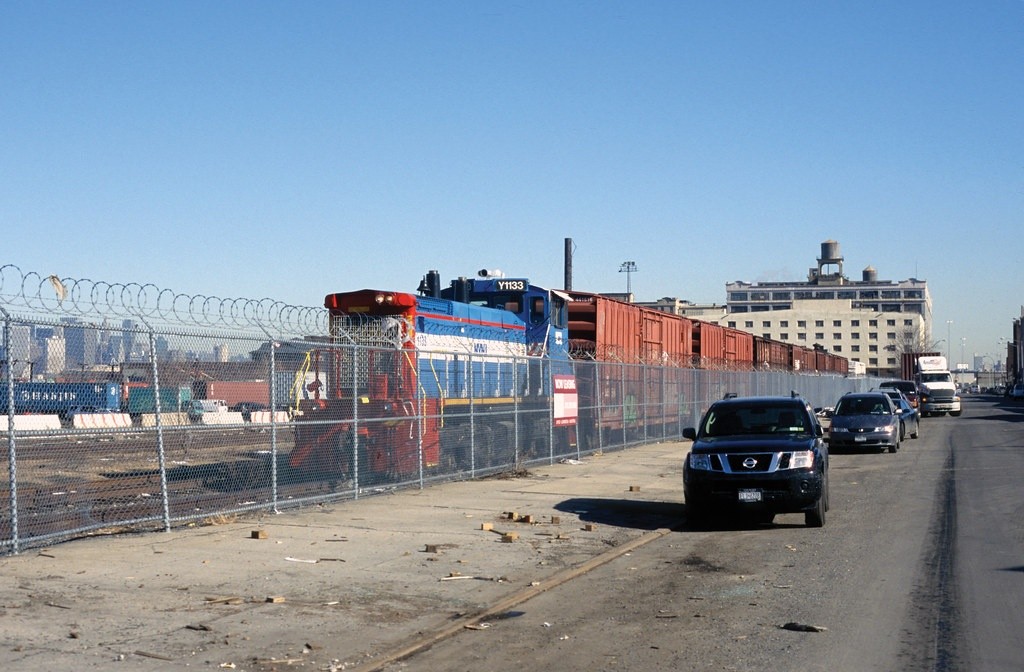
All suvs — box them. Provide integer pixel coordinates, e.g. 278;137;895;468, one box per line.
682;389;829;528
828;380;922;454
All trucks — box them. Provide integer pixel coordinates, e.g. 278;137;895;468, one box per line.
914;355;961;418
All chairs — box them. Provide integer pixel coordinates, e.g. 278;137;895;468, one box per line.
722;414;744;433
776;410;796;431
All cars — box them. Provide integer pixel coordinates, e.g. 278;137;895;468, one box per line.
993;383;1024;399
815;407;835;429
963;383;982;394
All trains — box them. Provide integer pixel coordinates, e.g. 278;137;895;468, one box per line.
286;267;849;480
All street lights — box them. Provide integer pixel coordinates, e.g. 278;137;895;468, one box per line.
617;261;639;294
944;319;953;369
961;337;966;364
